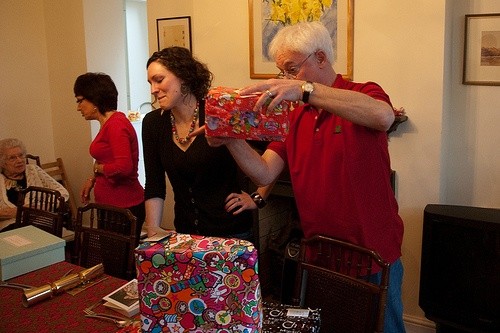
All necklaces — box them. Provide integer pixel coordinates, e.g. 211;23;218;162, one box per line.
170;100;199;145
100;110;116;125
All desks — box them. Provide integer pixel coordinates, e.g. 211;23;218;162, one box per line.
0;262;140;333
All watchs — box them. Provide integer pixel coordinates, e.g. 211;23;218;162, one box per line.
300;80;314;104
94;164;99;177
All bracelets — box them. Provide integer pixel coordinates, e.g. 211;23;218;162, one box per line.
250;191;267;209
88;178;95;181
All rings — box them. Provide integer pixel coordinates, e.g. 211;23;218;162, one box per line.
266;89;272;97
237;197;241;201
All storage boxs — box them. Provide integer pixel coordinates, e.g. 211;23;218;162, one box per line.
133;235;265;333
0;224;66;281
204;85;292;142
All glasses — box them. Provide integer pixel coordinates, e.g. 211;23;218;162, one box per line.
77;96;86;104
0;152;26;162
278;51;314;80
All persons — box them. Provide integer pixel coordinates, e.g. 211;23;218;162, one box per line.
73;71;145;282
140;46;262;245
188;22;405;333
0;138;70;219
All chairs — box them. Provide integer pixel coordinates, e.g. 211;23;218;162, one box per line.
293;235;391;333
42;157;79;226
15;186;65;237
73;203;139;280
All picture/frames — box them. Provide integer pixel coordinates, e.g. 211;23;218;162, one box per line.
248;0;354;82
156;16;192;54
462;13;500;86
